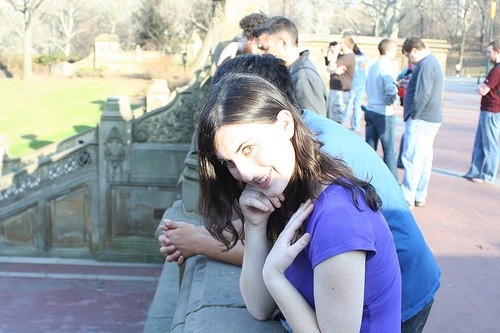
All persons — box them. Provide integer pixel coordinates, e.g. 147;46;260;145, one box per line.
462;38;500;183
158;53;441;333
397;60;417;172
240;12;269;55
193;73;403;333
322;33;368;127
455;62;462;78
363;39;402;188
400;37;444;209
253;16;329;121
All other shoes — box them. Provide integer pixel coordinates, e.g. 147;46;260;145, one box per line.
415;200;424;207
472;177;485;184
407;199;415;209
